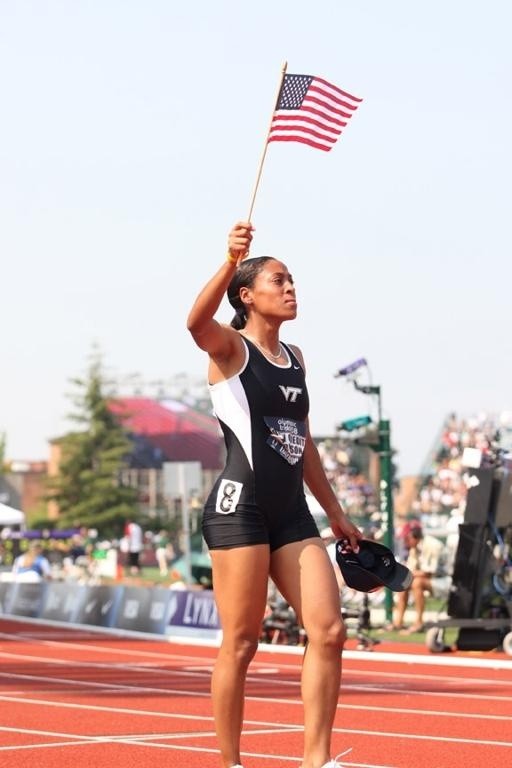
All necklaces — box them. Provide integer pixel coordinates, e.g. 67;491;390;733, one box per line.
242;331;286;360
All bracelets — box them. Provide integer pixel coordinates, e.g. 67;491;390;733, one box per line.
226;248;250;262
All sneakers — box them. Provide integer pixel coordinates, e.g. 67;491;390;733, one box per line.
229;762;346;768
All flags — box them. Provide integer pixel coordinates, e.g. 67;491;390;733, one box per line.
268;72;363;153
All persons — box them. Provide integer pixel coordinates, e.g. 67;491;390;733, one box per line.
256;409;510;652
0;518;208;596
185;219;370;767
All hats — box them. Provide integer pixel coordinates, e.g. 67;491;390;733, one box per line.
334;538;414;593
